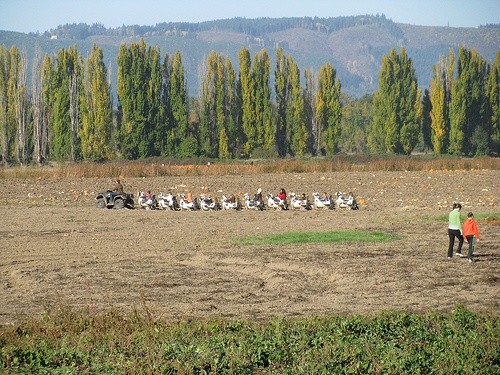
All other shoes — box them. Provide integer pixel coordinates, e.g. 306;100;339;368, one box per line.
456;253;464;257
466;258;473;262
448;257;453;261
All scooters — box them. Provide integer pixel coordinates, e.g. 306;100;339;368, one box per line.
95;191;135;210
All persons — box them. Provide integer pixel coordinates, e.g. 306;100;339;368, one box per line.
163;192;174;202
299;193;306;201
184;192;193;203
252;188;263;207
276;189;287;202
108;180;124;206
463;212;479;262
320;193;329;202
447;203;465;258
142;190;152;200
344;192;354;205
